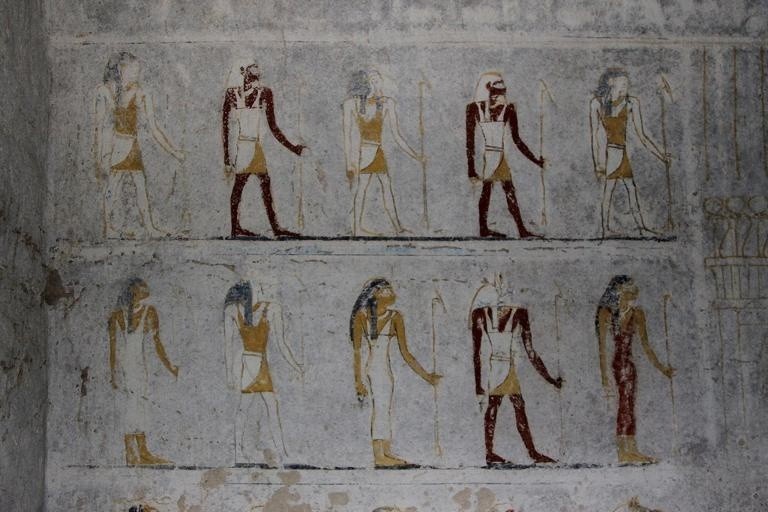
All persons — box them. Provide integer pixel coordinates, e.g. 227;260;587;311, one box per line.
338;65;428;239
106;277;181;467
94;49;188;241
589;66;672;240
465;271;568;469
223;277;307;464
462;73;548;237
590;272;681;465
346;275;442;469
217;53;311;242
